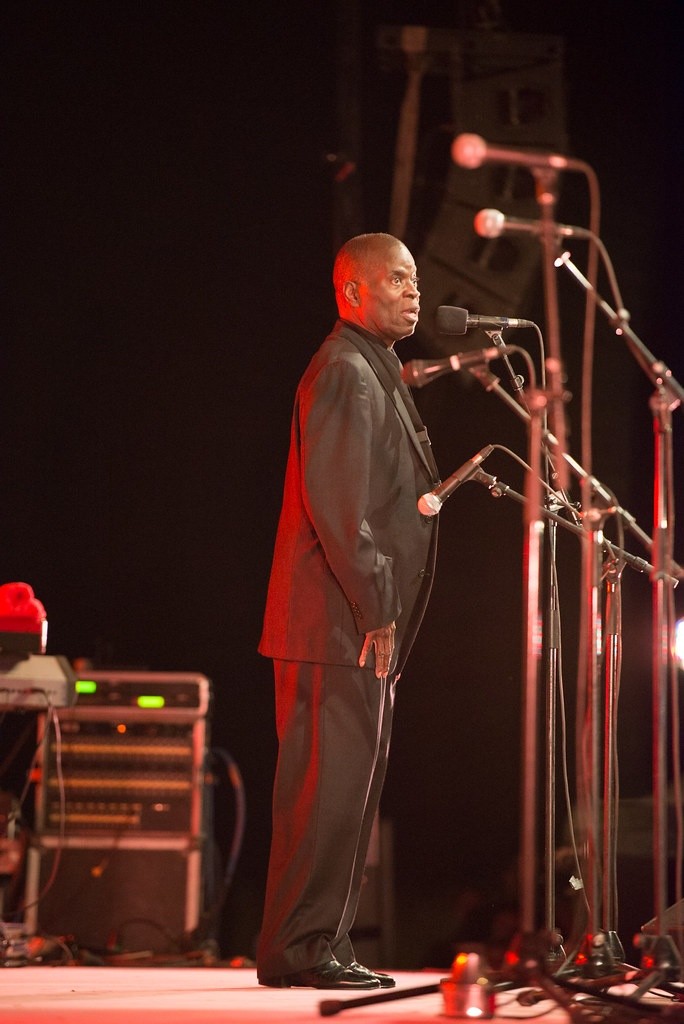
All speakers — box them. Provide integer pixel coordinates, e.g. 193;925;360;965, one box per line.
642;899;684;986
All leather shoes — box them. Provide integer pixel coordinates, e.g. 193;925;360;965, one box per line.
258;958;397;990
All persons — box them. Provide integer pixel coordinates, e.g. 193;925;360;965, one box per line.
256;235;438;990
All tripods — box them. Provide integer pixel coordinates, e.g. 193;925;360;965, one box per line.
321;163;684;1024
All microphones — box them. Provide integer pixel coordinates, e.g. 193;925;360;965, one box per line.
434;305;534;334
451;134;585;172
473;209;594;239
419;445;493;517
402;343;513;386
